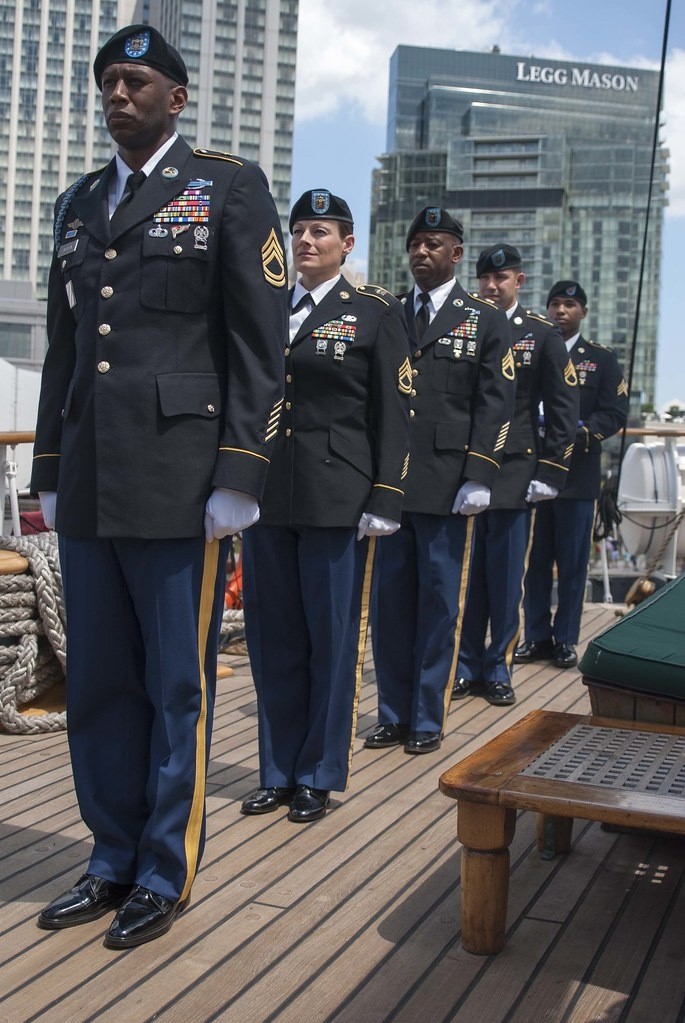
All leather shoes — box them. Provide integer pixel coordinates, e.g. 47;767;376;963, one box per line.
404;731;445;753
364;724;409;747
289;785;330;821
483;682;516;705
553;642;578;668
105;884;192;948
514;640;555;663
38;873;133;928
450;678;476;699
241;787;291;813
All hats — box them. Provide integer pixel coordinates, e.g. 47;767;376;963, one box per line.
406;206;464;253
288;189;354;236
93;24;188;91
476;244;522;279
546;281;587;310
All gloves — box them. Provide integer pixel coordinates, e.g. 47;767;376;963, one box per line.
525;480;560;504
451;481;491;516
38;492;57;531
204;489;260;542
357;513;401;542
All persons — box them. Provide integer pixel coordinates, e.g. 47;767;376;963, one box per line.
37;24;291;948
450;244;579;705
240;189;411;821
512;281;628;667
363;208;516;754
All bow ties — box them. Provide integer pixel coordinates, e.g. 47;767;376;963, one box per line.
292;293;316;315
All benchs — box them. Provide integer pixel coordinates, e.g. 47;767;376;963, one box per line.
580;577;685;833
438;710;685;957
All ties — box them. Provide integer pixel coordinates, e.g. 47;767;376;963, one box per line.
415;292;431;341
110;171;147;221
